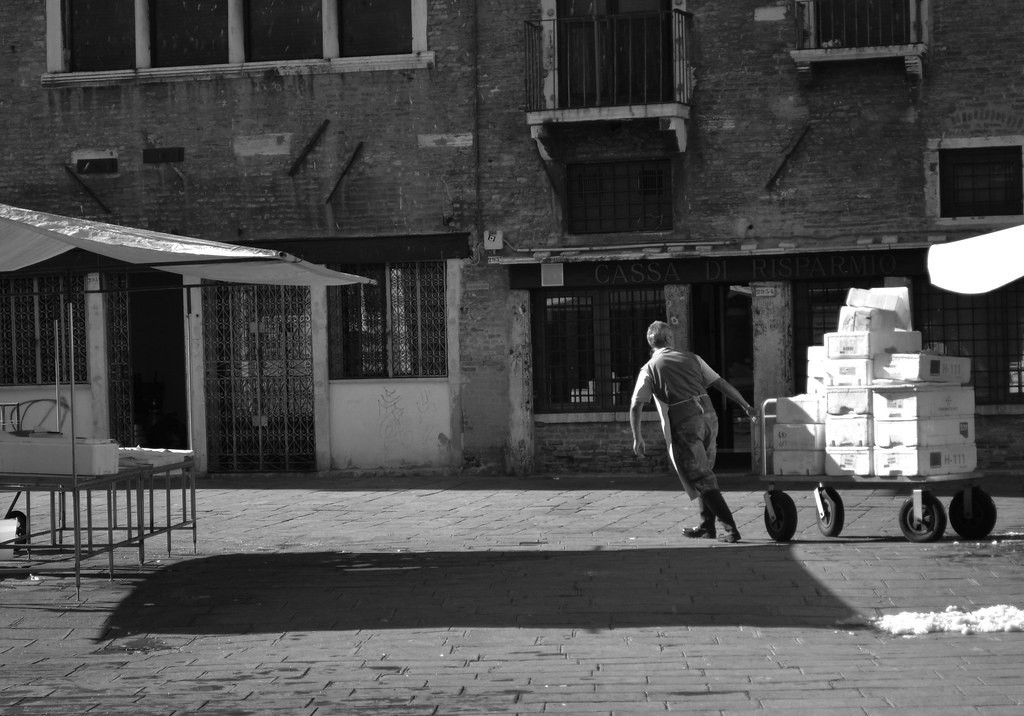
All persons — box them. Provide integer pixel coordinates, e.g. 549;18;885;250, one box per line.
629;321;757;541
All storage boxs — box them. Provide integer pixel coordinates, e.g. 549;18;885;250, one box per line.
773;284;980;476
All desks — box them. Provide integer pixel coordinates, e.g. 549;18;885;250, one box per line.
0;456;195;603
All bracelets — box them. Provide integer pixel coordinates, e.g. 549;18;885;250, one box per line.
744;405;750;410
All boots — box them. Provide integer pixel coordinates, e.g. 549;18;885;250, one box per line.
700;489;741;542
682;496;716;539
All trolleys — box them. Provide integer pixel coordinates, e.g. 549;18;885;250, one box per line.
758;397;999;545
